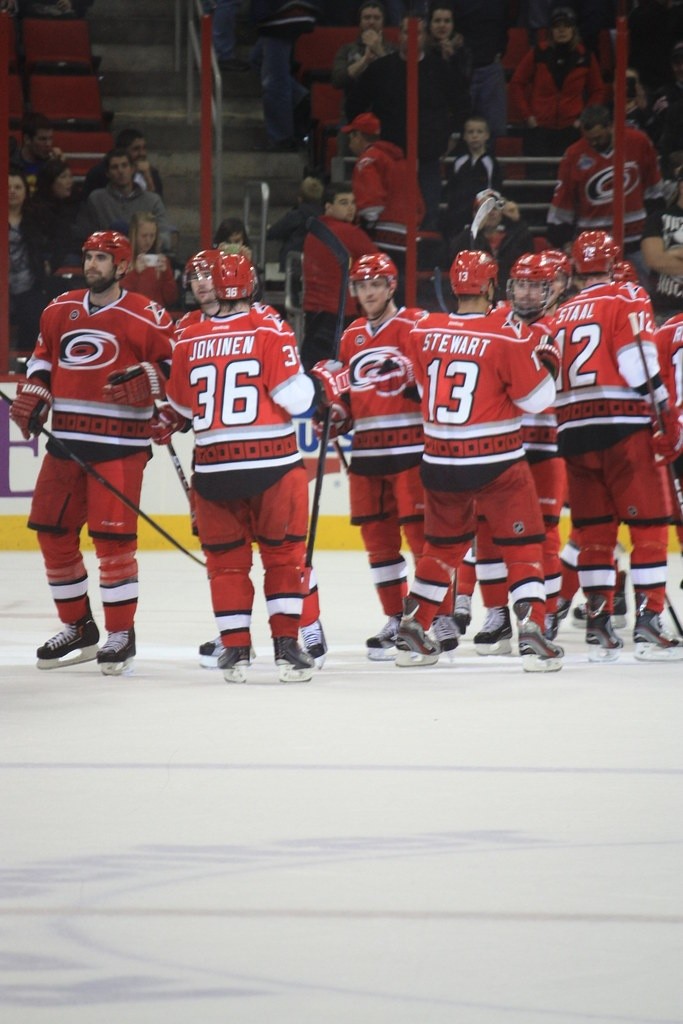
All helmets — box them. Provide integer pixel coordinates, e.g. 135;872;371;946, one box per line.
83;229;133;266
452;230;640;318
183;248;261;303
348;252;396;298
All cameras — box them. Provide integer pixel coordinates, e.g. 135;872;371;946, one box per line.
494;200;504;209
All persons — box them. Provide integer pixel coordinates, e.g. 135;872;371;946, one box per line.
0;0;683;682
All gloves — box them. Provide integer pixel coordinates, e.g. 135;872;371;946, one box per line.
529;323;562;379
150;403;191;444
649;403;683;468
373;355;417;395
311;399;355;445
104;362;166;407
310;357;350;406
11;377;54;442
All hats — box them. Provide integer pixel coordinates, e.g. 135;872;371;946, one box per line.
550;8;577;24
341;112;381;135
472;187;500;206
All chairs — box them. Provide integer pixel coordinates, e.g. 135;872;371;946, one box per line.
299;16;615;205
9;10;116;190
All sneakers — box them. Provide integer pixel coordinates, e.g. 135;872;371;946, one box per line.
36;597;100;671
198;634;256;670
301;617;330;670
95;621;137;676
367;588;683;674
216;646;250;685
272;636;315;683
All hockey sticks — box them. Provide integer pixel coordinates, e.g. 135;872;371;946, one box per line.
628;311;683;510
1;390;207;567
469;197;496;248
434;264;451;313
304;215;354;567
165;440;195;497
